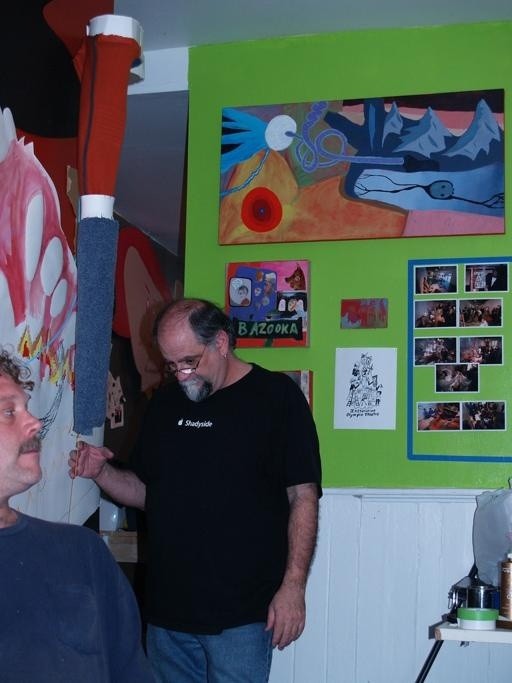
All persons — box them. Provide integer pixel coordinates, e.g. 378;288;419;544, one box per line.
68;298;324;683
0;350;155;683
483;270;500;291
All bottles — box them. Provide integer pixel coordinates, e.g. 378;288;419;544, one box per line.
498;553;512;619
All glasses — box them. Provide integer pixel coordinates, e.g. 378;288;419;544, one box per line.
165;343;208;375
